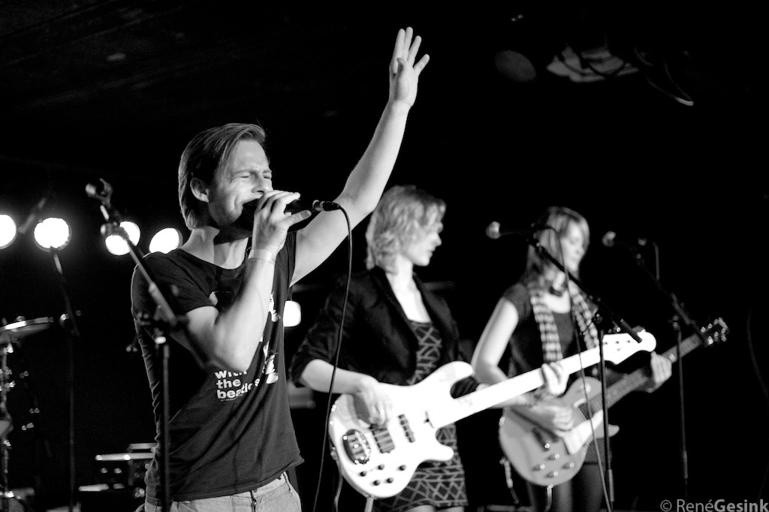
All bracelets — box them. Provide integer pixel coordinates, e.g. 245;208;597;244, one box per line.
245;245;280;263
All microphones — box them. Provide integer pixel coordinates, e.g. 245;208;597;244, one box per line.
602;231;647;247
263;199;341;215
485;221;551;239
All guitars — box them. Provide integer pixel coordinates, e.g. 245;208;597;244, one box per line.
497;318;729;486
327;323;657;499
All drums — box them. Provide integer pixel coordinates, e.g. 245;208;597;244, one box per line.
2;488;35;511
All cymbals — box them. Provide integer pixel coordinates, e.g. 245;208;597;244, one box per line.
1;316;53;345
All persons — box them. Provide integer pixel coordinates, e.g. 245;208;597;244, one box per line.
470;202;676;512
289;185;573;511
129;22;433;512
